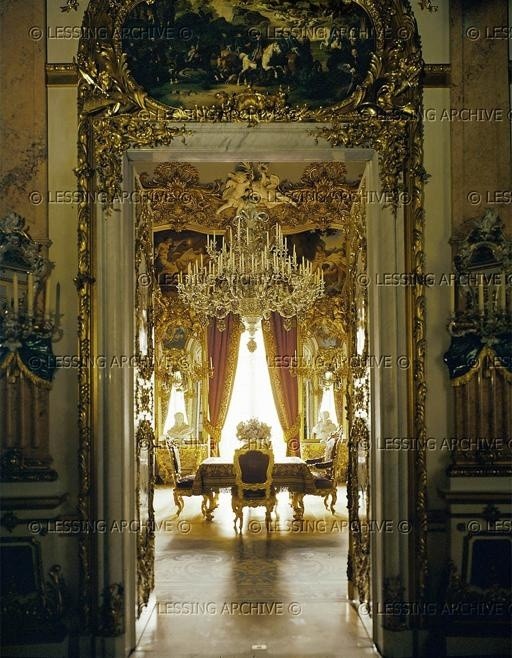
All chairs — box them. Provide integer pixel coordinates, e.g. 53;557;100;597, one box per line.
160;427;344;526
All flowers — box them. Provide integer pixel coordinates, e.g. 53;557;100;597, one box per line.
235;416;273;442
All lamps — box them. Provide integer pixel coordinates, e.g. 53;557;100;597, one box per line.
172;182;327;359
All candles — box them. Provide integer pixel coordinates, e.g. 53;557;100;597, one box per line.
445;267;508;328
11;268;53;328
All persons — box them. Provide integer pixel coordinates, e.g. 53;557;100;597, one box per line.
251;173;284;209
216;170;255;217
167;412;190;437
